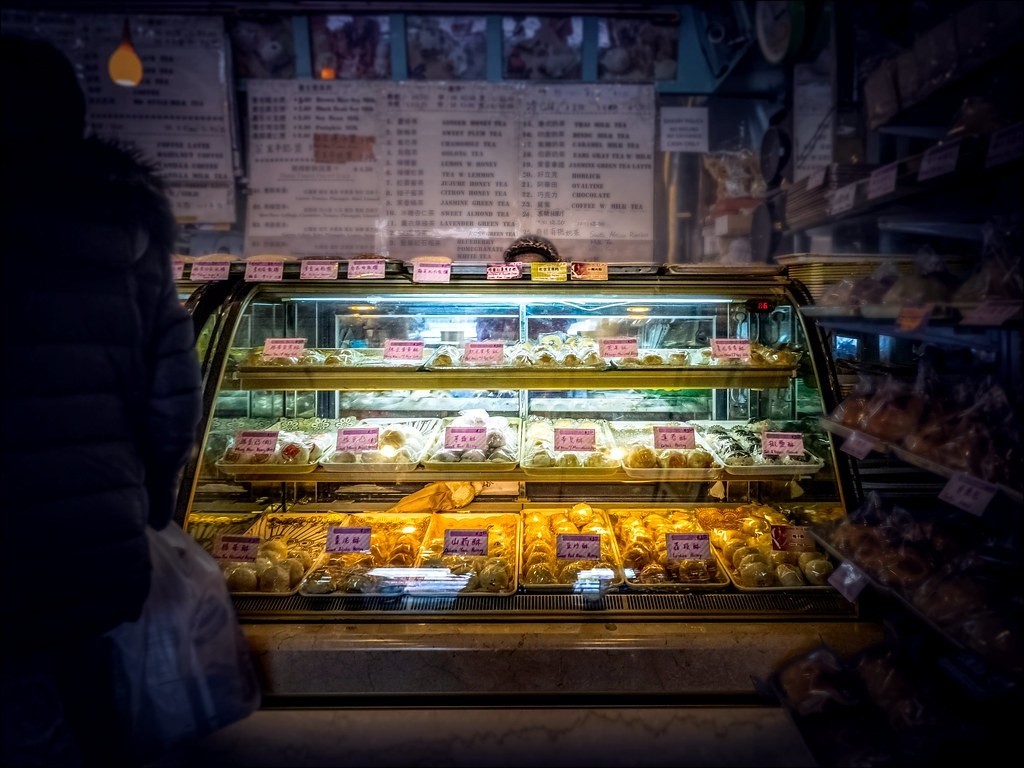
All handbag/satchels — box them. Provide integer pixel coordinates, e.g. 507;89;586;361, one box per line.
110;521;260;754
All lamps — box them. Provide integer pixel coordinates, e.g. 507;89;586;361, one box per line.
107;0;144;88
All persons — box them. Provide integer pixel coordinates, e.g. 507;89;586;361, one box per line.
476;236;576;398
0;25;201;768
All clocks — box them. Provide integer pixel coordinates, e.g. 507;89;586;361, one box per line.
754;1;826;63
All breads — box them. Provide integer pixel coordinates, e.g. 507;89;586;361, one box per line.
835;390;1024;655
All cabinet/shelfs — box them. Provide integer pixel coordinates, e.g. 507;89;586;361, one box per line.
178;120;1024;768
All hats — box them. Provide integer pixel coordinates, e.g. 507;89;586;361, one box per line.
503;235;558;261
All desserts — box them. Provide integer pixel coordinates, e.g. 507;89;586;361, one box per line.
215;349;833;590
